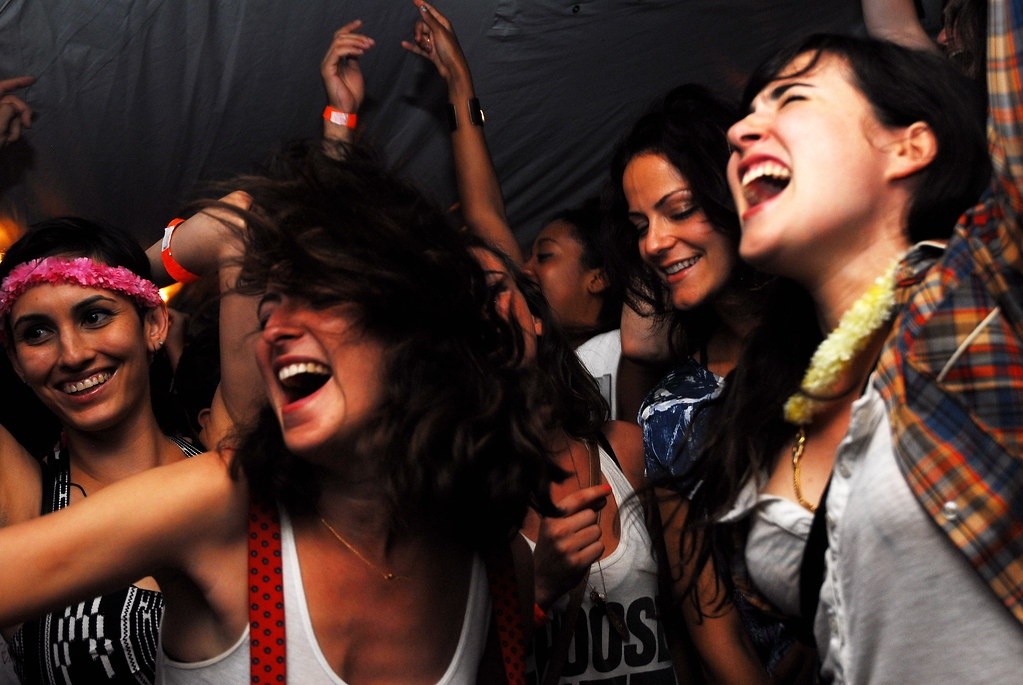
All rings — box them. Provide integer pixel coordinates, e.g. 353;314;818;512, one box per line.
427;37;430;43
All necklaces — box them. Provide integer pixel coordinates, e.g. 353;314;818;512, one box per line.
791;426;818;514
530;438;607;603
319;507;425;582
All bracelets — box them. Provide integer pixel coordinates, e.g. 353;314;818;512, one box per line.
443;97;484;128
323;106;357;129
161;218;201;284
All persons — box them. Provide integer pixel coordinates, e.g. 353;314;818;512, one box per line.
0;0;1023;685
